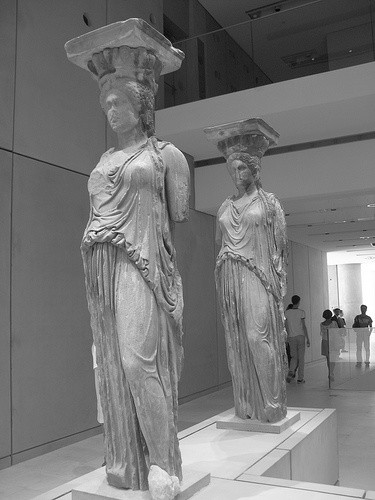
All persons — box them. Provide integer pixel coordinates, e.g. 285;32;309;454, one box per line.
213;151;289;422
78;49;190;491
276;293;309;384
319;308;348;383
351;304;373;367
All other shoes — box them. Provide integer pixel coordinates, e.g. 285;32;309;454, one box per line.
297;379;305;384
286;370;295;383
